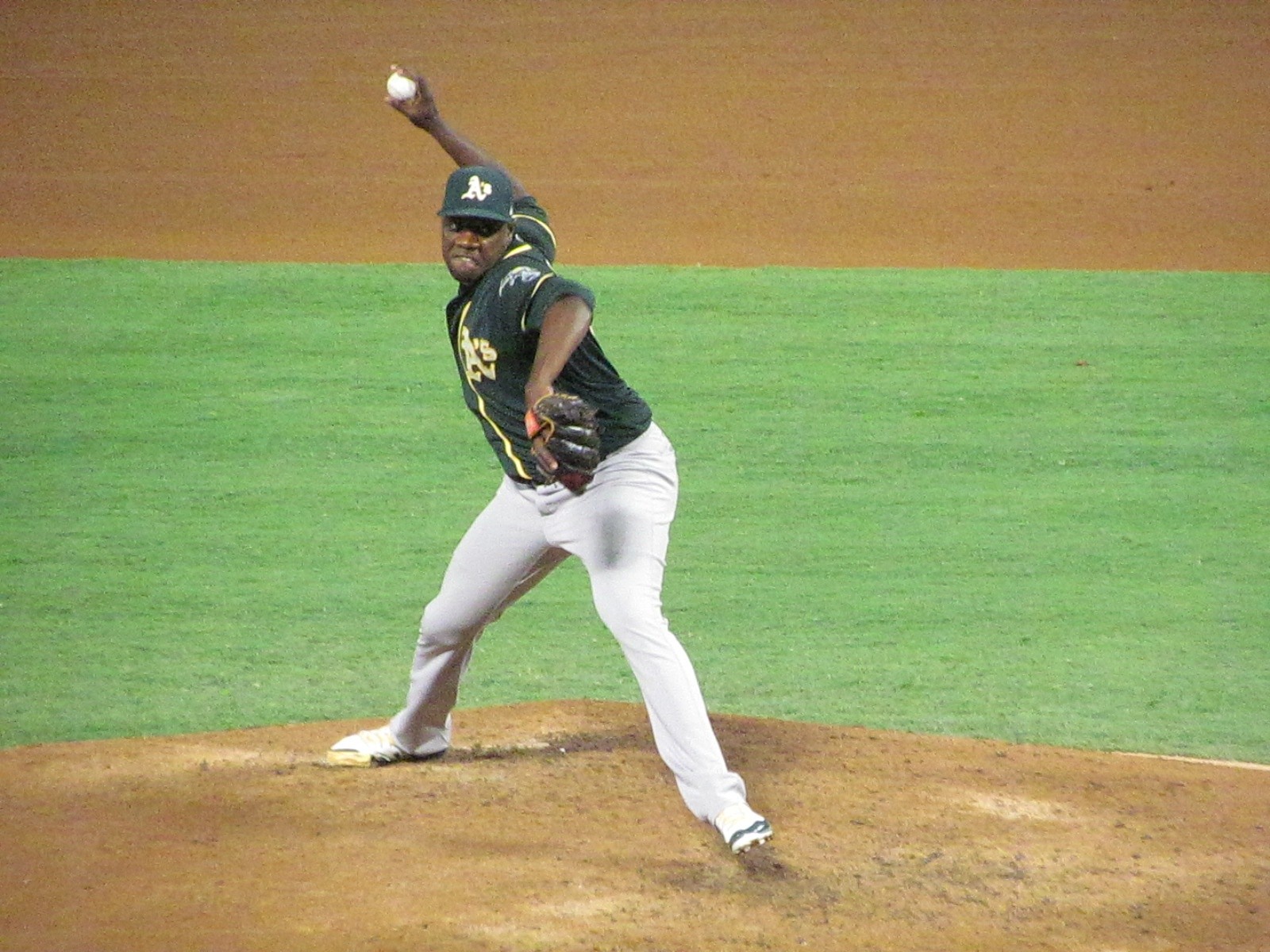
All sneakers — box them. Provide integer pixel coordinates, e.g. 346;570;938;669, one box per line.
714;803;774;854
327;725;446;769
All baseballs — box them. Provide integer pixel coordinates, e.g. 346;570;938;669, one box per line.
387;72;418;101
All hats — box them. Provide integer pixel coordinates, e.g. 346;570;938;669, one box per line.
437;166;514;222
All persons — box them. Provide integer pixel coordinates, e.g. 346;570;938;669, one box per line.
332;64;771;856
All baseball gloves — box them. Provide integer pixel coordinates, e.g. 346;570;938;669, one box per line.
535;390;600;497
